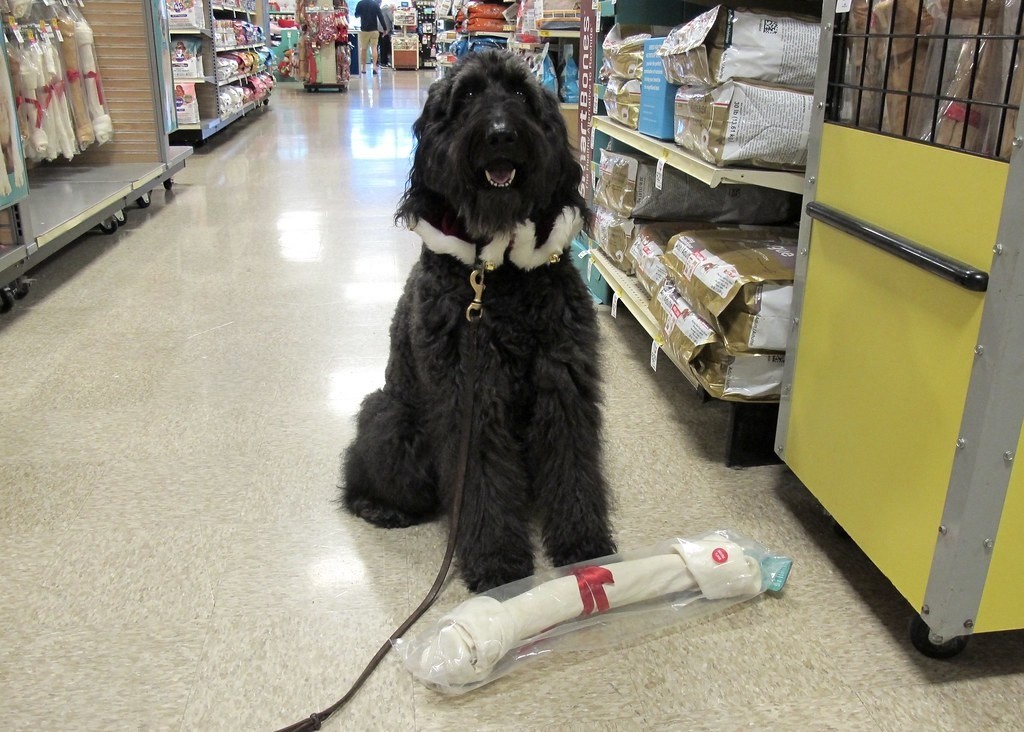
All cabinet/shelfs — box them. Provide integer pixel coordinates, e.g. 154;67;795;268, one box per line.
584;115;806;469
170;28;221;141
208;0;271;133
534;28;580;164
435;15;455;69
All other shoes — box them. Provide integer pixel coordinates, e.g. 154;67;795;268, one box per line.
381;63;388;69
361;71;366;73
373;69;378;74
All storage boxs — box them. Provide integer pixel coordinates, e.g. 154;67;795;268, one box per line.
638;36;678;140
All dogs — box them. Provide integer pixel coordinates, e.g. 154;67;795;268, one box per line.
337;47;616;600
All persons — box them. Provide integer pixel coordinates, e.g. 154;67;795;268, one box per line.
355;0;388;74
378;0;396;69
